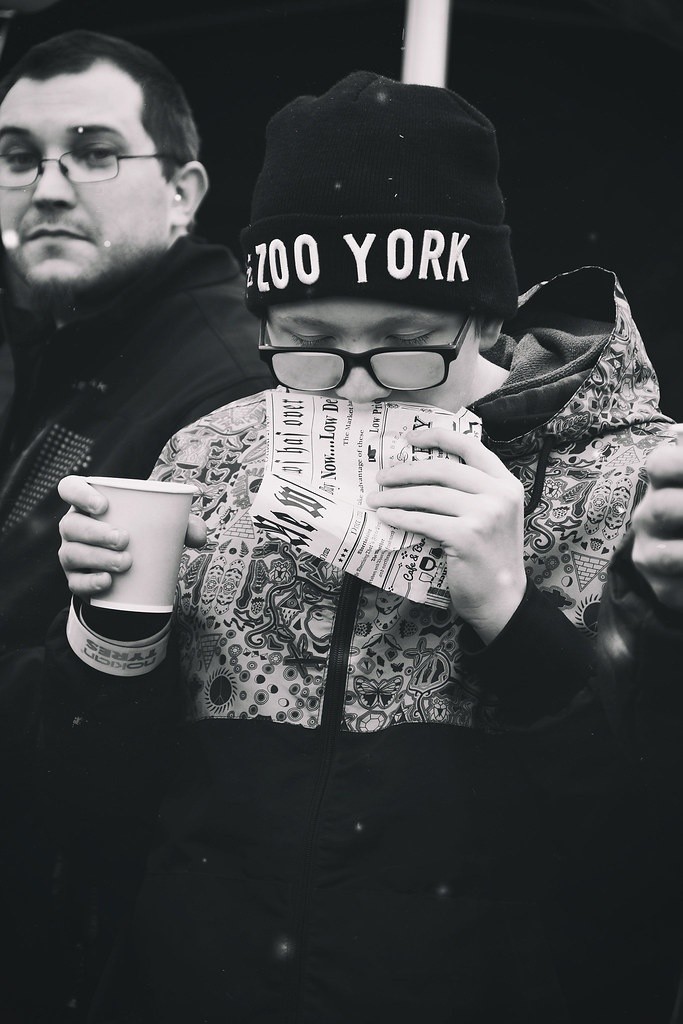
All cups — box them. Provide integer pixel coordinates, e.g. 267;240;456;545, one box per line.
84;476;197;613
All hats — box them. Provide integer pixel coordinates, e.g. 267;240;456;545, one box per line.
237;70;519;321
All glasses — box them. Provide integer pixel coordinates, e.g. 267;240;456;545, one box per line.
257;310;474;391
0;148;176;188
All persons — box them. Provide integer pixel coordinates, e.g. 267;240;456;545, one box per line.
0;29;280;1024
57;72;683;1024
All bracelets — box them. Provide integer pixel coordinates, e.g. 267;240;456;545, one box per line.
67;598;170;678
